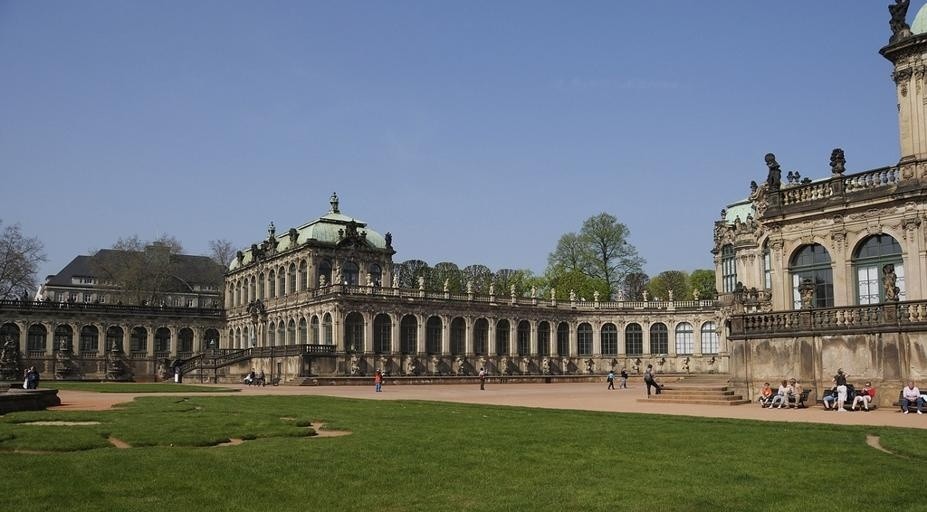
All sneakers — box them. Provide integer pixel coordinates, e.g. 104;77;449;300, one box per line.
824;408;870;412
904;410;924;414
762;404;798;409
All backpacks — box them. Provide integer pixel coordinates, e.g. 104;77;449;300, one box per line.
645;369;651;382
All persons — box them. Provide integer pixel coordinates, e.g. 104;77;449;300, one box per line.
619;367;629;389
479;367;488;390
23;366;39;389
249;367;266;387
375;369;383;392
823;367;875;413
758;378;804;409
607;371;617;390
644;364;661;398
903;380;923;415
174;363;180;383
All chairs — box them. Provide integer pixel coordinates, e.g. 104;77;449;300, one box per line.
762;388;926;413
